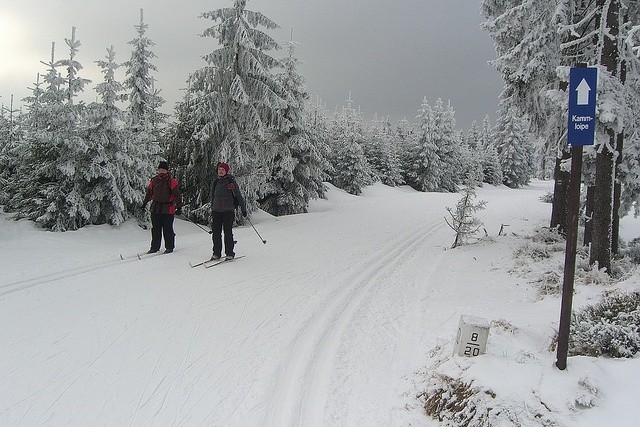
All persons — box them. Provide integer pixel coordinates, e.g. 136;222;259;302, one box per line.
210;163;247;260
141;162;181;253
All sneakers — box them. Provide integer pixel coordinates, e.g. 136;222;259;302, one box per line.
147;249;157;254
163;249;173;254
225;256;233;261
211;255;218;261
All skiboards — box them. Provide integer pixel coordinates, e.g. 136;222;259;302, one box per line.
118;246;185;260
187;252;246;268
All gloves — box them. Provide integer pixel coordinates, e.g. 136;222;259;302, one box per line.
140;204;145;211
242;211;247;217
175;208;182;215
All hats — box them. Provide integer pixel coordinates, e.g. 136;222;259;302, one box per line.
217;162;229;172
158;161;169;170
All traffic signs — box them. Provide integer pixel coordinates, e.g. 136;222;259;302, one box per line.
568;66;599;147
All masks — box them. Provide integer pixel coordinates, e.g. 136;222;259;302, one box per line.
158;173;167;177
